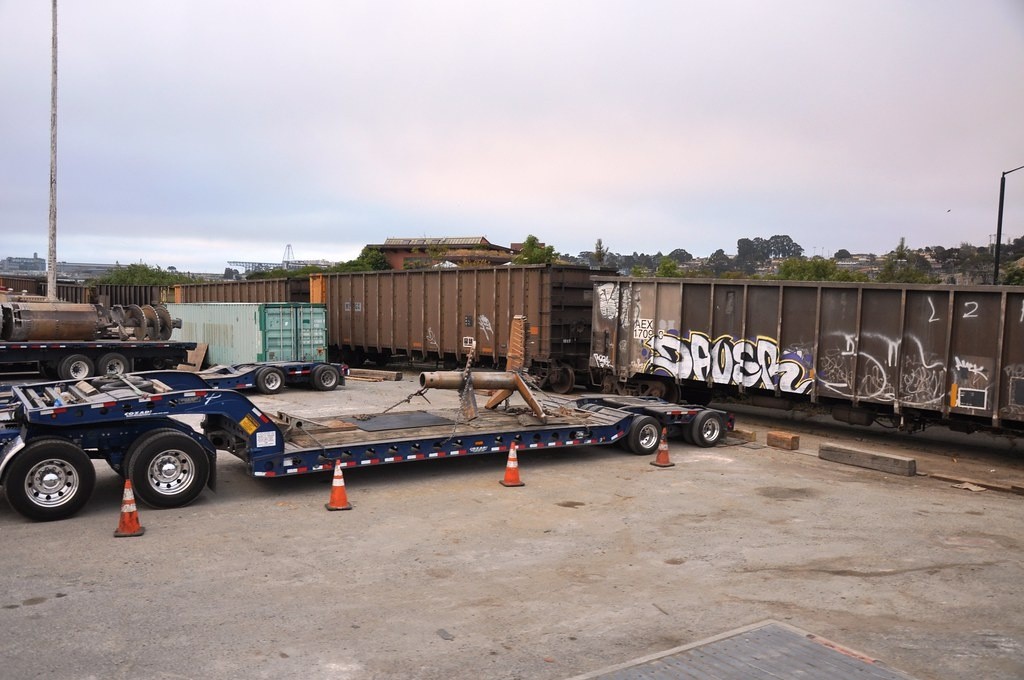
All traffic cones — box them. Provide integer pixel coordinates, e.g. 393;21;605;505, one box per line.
649;427;677;467
111;479;145;538
499;441;535;490
324;460;354;511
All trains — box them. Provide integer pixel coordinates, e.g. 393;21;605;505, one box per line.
0;259;1024;434
